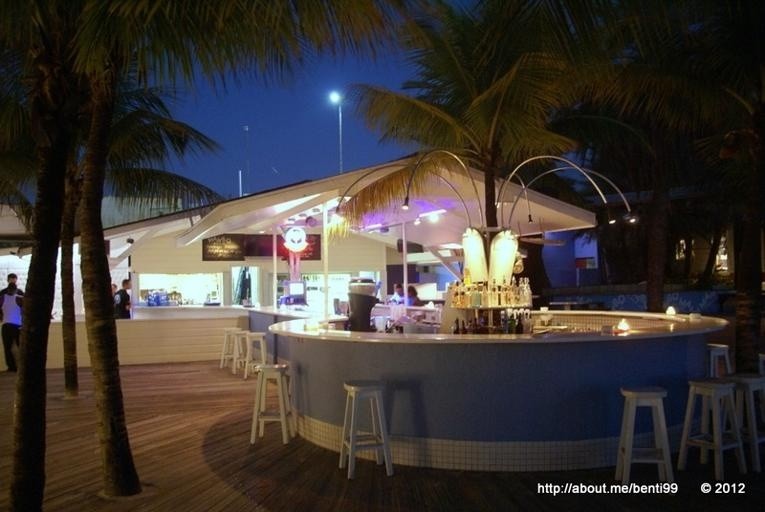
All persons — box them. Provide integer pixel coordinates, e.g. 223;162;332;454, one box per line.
0;273;24;306
111;285;119;298
1;282;26;372
388;285;405;305
399;285;420;306
115;277;133;318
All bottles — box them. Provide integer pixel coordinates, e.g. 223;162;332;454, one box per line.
450;276;534;334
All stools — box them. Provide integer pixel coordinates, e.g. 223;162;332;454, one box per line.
615;344;765;485
338;380;394;480
221;327;296;444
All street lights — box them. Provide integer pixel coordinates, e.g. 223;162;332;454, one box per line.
330;92;347;173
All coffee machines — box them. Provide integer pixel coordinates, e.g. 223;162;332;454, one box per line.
348;279;380;334
